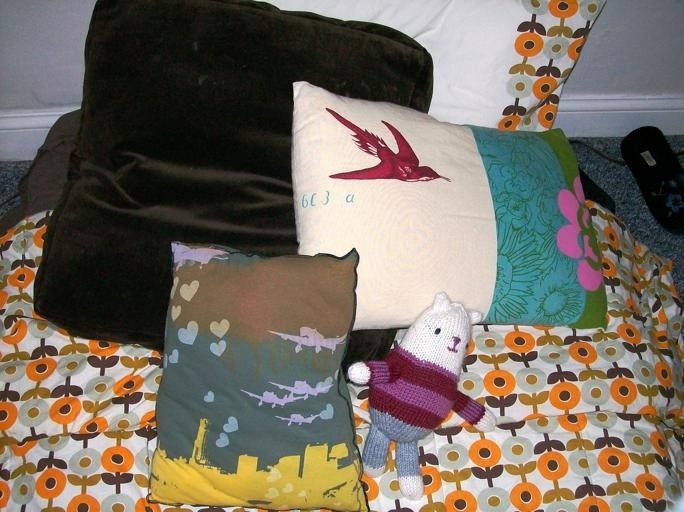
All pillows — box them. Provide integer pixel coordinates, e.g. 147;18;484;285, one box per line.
259;0;604;134
292;79;607;332
147;242;369;511
33;0;432;352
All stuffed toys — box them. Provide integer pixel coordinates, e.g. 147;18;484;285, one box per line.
347;291;497;501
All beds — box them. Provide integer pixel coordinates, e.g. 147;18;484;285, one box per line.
0;135;684;511
